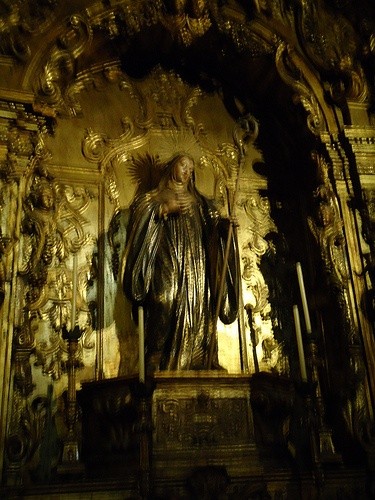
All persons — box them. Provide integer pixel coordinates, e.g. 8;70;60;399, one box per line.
119;152;240;373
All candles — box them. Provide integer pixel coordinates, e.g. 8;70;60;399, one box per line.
292;305;308;382
70;253;78;331
295;262;311;334
